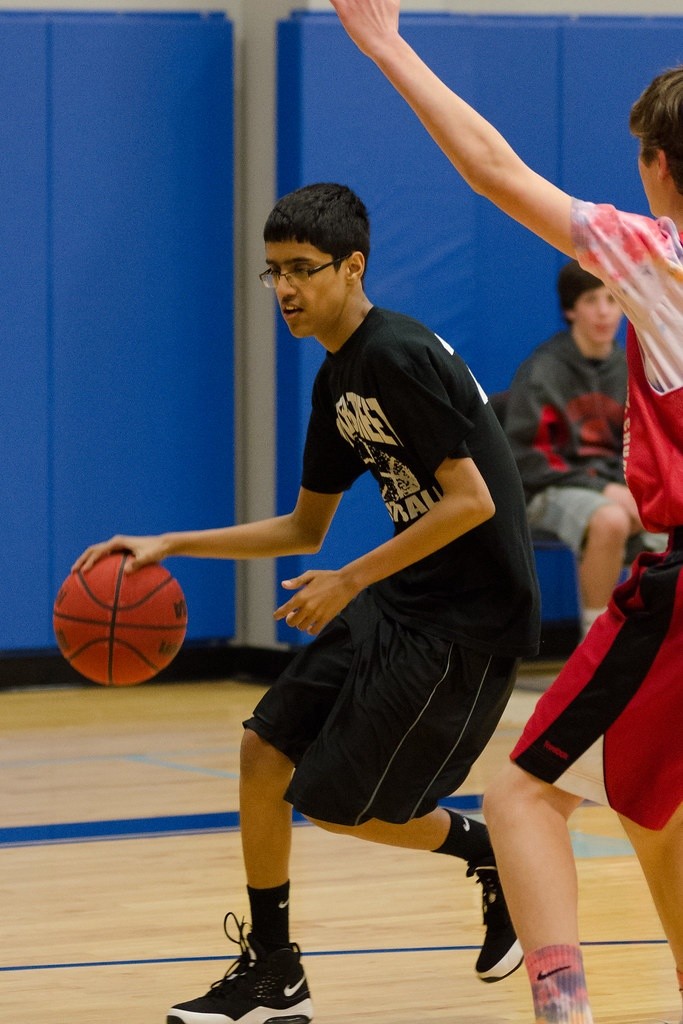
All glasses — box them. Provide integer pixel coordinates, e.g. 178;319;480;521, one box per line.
259;253;352;288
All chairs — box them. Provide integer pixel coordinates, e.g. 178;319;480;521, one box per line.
483;392;657;564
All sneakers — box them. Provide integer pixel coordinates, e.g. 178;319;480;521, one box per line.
167;912;314;1024
466;853;524;983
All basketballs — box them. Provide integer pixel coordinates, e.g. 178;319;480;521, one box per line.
52;552;188;687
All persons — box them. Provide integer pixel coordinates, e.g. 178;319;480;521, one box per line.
71;184;540;1024
500;256;639;642
330;0;683;1024
